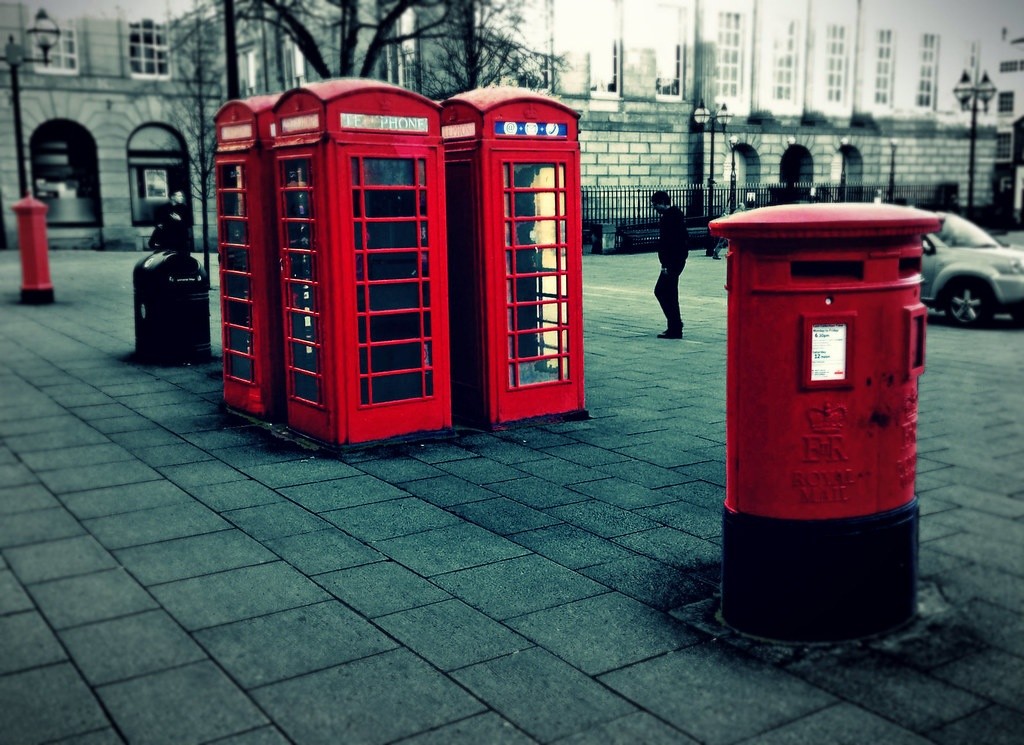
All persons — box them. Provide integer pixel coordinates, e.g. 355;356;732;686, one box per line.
651;191;688;340
151;185;192;257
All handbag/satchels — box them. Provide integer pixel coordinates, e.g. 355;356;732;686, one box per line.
149;227;165;251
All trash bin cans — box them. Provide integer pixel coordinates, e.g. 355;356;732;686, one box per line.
134;252;215;367
592;223;618;255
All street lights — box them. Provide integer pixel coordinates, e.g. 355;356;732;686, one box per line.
951;71;997;207
1;8;63;304
693;99;735;219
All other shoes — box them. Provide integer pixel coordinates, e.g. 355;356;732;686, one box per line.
713;257;721;259
657;329;682;339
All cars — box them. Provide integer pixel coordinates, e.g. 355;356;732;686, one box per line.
921;211;1023;327
